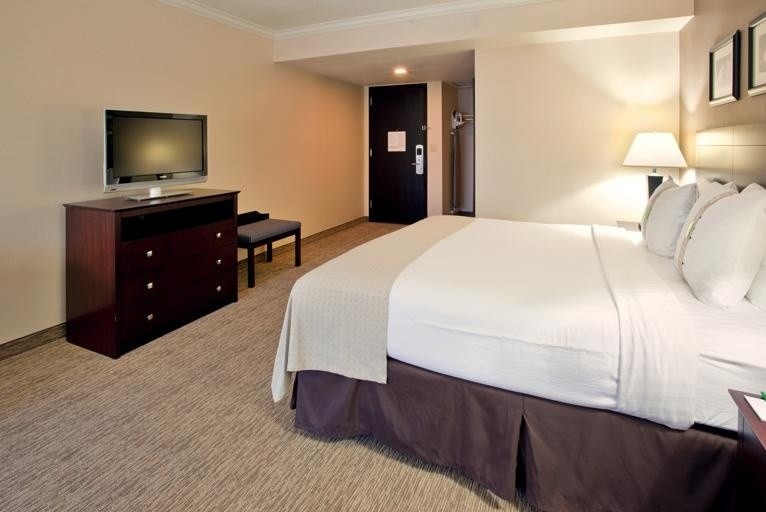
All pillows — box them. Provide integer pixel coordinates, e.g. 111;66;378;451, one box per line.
673;182;766;308
640;180;700;255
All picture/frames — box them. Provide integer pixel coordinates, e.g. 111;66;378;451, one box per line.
747;13;766;99
708;30;740;105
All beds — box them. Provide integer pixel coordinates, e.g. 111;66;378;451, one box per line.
293;215;766;512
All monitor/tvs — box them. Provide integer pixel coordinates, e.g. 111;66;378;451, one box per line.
101;109;208;202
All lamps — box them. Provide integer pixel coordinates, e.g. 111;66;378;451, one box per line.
621;130;689;201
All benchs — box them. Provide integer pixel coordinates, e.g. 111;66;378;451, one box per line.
237;211;300;289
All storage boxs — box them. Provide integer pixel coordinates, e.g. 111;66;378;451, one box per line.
64;192;238;359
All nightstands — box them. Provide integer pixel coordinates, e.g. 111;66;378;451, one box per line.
727;388;766;512
